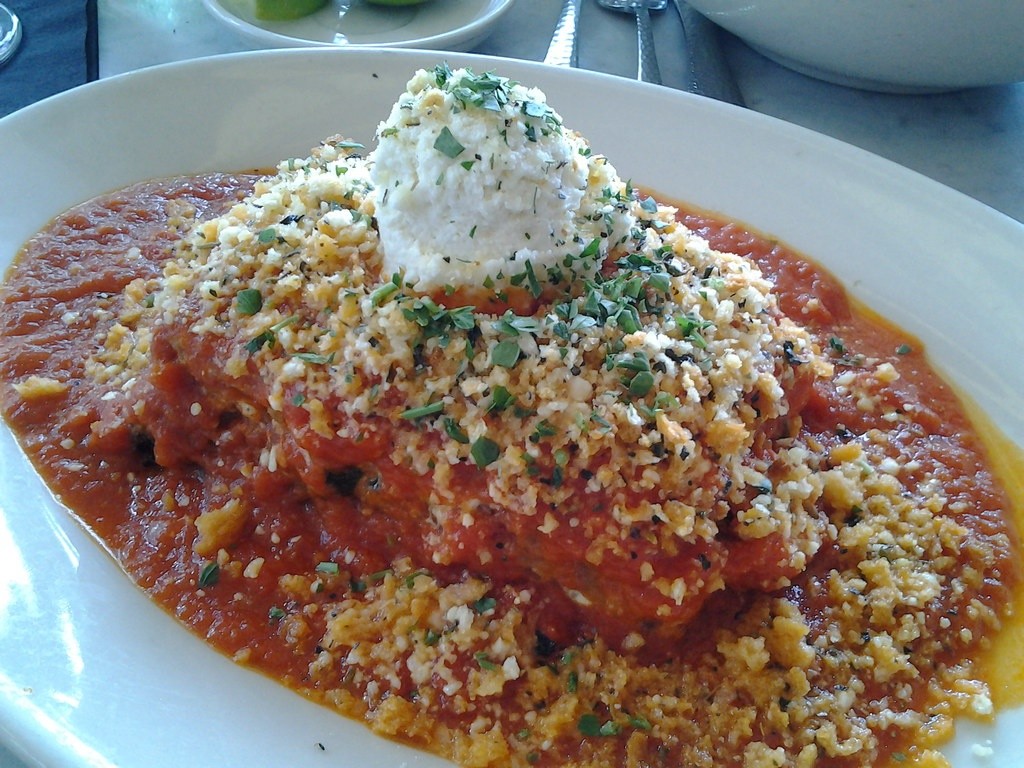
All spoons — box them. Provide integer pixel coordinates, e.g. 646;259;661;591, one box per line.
598;0;667;86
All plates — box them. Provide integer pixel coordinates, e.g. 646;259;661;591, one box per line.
0;47;1024;768
205;0;513;51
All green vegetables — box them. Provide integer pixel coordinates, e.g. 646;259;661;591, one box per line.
198;60;854;740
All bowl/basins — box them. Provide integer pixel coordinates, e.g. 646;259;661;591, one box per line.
688;0;1024;94
